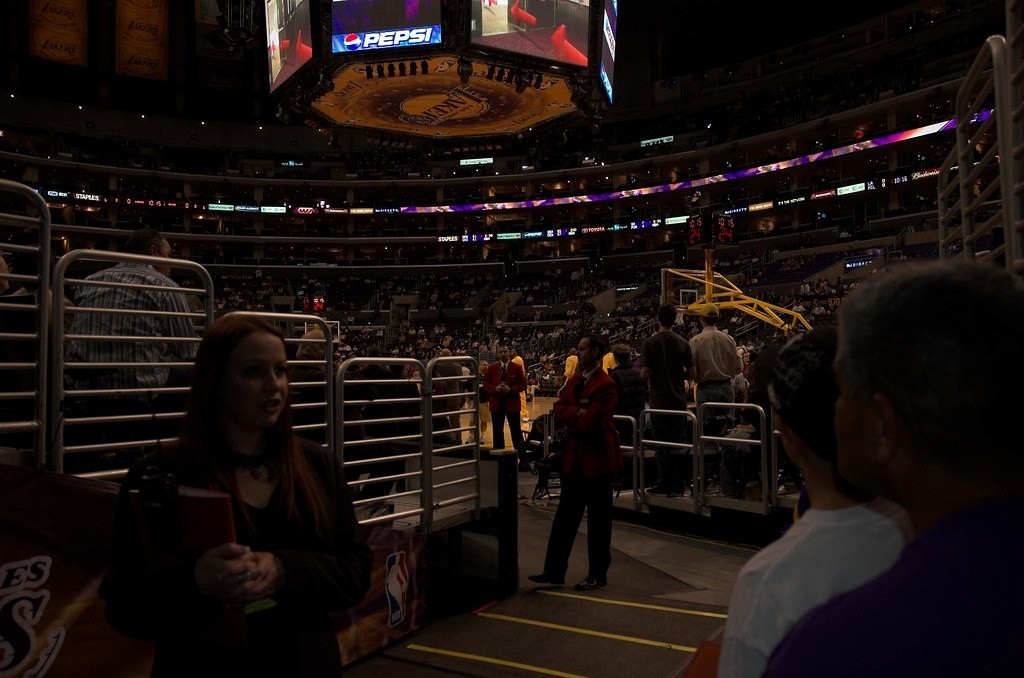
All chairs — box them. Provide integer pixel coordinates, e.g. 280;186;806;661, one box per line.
615;418;731;497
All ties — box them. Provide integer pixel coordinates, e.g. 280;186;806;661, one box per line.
502;364;506;381
578;377;586;391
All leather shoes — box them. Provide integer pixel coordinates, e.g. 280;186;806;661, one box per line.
574;576;607;590
528;569;565;585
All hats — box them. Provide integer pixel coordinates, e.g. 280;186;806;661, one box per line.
697;302;720;317
298;330;325;358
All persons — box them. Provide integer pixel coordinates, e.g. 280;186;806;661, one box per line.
638;305;696;497
98;316;375;678
758;262;1023;678
717;327;914;678
62;229;200;466
0;61;1023;496
483;346;529;468
688;303;738;482
527;333;625;590
608;344;648;489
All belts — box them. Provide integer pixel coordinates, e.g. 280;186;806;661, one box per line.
706;379;731;384
